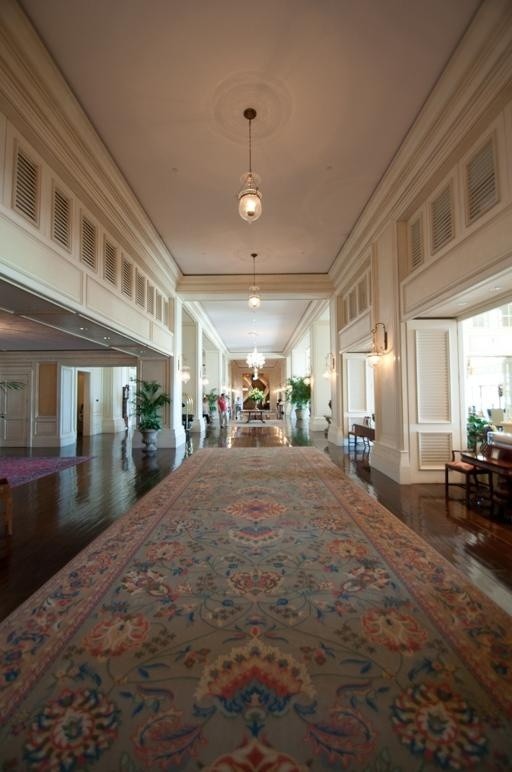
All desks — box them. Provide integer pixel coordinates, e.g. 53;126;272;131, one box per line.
460;449;512;508
241;410;271;424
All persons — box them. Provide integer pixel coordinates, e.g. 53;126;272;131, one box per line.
235;397;241;417
218;394;229;428
203;398;210;425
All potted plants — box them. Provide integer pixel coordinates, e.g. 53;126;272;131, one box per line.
287;375;312;420
128;376;171;455
203;388;220;417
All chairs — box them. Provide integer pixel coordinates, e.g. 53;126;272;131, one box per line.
2;476;17;537
441;431;494;506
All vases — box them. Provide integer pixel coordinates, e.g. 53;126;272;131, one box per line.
251;398;261;410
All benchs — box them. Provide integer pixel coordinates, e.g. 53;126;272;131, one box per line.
347;425;377;448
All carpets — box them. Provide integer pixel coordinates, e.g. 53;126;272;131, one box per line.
0;453;100;486
1;446;512;771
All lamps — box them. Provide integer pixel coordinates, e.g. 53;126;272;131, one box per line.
244;332;266;379
202;362;210;387
367;320;394;371
235;100;267;221
323;350;338;380
181;365;191;385
246;250;264;310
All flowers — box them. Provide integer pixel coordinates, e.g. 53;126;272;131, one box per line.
248;386;264;401
